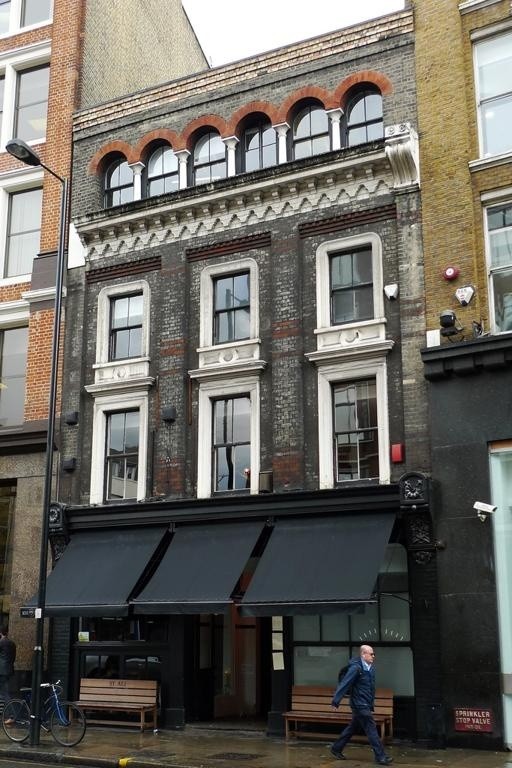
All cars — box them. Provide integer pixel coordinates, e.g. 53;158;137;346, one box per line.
84;653;158;679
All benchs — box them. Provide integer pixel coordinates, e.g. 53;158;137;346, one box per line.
281;686;393;746
69;679;160;733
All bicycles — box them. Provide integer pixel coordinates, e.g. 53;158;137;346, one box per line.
0;680;88;747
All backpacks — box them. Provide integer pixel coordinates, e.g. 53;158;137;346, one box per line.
338;661;362;696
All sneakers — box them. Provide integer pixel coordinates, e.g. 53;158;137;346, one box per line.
330;747;346;760
376;755;395;765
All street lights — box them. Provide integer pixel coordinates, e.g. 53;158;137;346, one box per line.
4;135;69;747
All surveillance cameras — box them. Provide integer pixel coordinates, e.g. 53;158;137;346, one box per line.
473;502;498;523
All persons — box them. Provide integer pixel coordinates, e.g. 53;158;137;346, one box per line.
329;645;393;764
0;628;16;724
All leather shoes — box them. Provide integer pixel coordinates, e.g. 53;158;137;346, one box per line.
4;719;14;724
